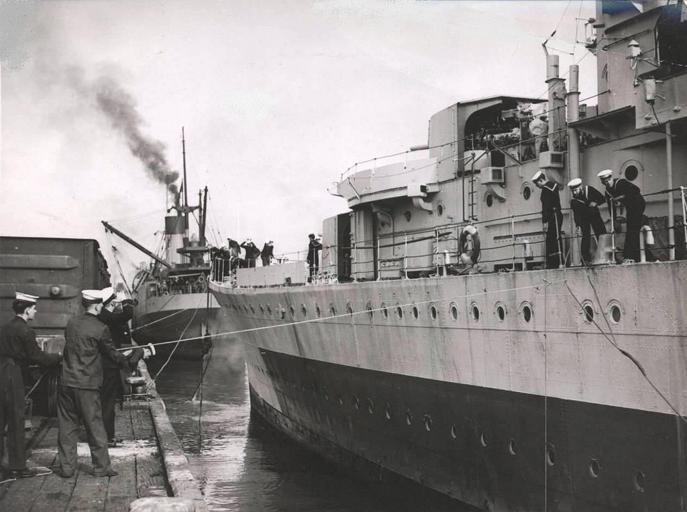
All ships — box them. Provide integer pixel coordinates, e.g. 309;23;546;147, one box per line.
105;125;221;361
208;0;686;512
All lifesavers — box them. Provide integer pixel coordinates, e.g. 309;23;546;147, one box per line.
458;225;480;268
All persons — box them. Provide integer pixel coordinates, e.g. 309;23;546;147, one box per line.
306;233;323;283
55;288;150;478
532;171;565;269
0;291;58;479
598;169;660;263
227;239;275;268
566;178;609;267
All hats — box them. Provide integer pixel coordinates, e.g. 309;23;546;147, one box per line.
597;169;612;179
148;342;156;356
567;178;582;188
81;289;102;303
531;171;545;182
15;292;40;303
102;287;117;305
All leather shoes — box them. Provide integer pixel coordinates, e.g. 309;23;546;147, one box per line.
9;468;36;478
107;470;118;477
